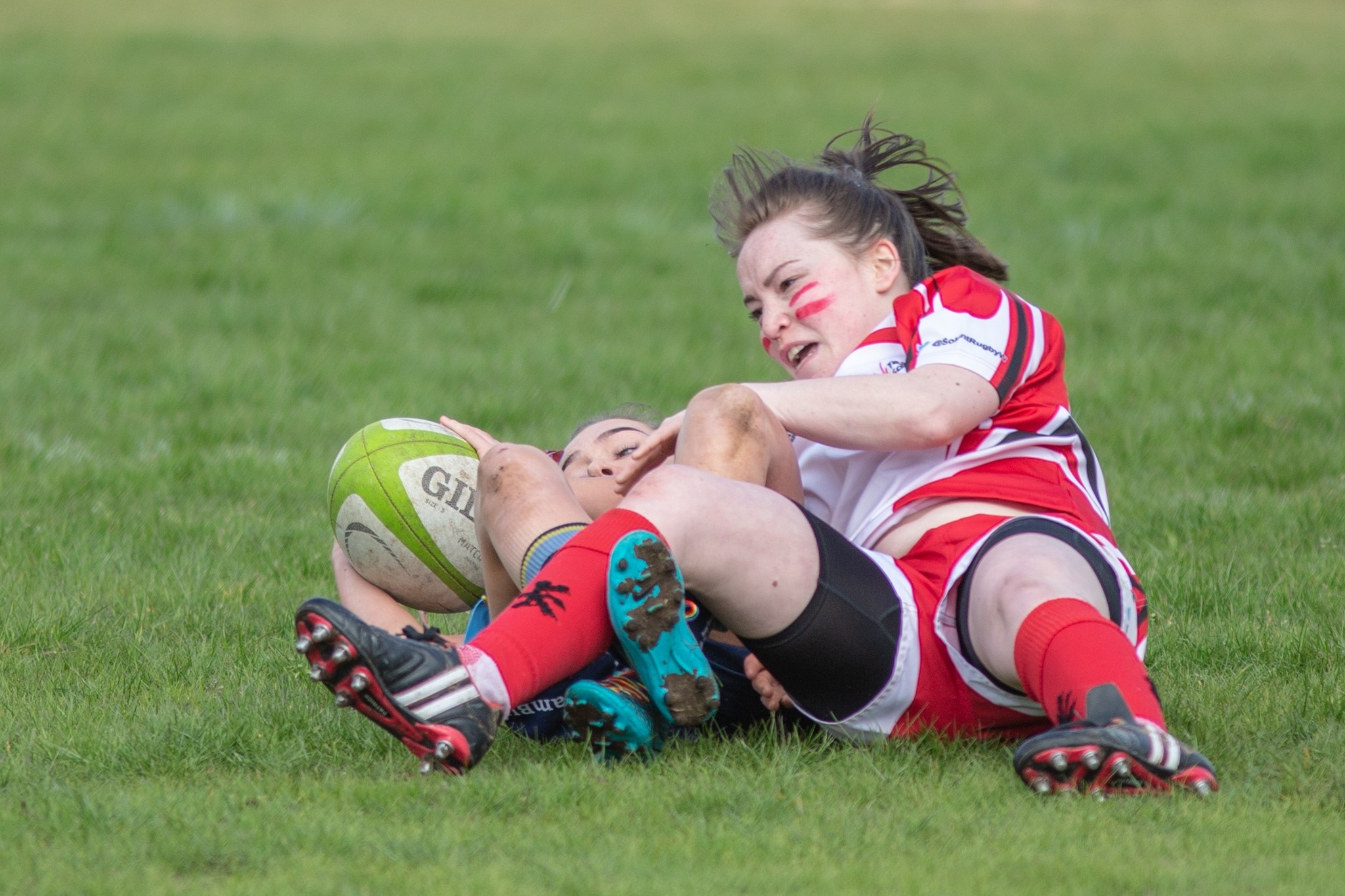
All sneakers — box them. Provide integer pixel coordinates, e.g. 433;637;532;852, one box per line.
291;597;505;777
562;668;661;764
1013;681;1218;801
605;529;723;731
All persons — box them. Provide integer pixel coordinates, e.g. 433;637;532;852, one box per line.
293;110;1218;798
329;401;794;764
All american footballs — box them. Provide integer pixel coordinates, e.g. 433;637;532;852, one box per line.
326;417;488;615
